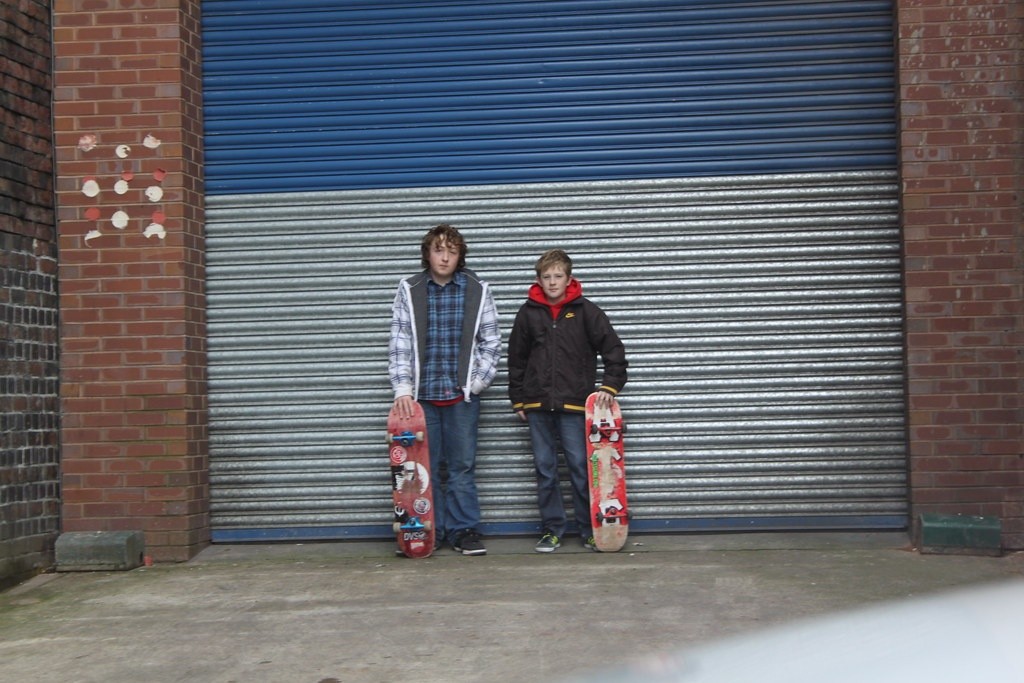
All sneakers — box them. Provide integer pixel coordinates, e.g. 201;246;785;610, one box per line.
584;536;601;551
535;530;560;551
454;531;487;555
395;538;443;554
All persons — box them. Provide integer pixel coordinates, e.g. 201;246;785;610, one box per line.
507;250;628;552
387;225;501;557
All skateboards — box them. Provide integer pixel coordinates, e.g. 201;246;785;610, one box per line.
387;398;436;560
585;392;632;552
